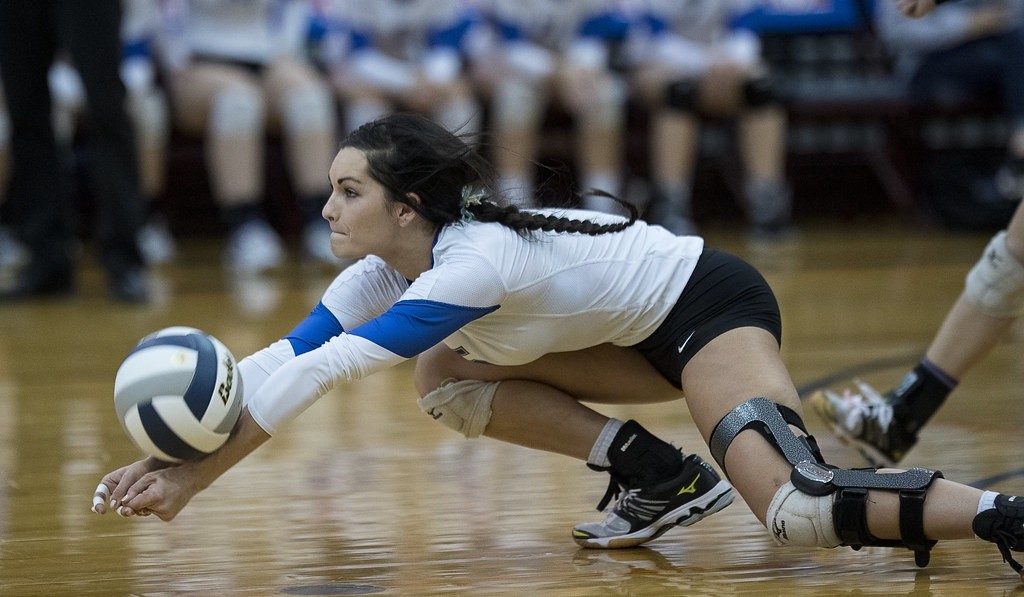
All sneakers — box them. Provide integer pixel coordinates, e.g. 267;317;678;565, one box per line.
811;379;920;467
573;451;732;548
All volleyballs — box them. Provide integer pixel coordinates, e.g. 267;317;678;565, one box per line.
114;326;243;464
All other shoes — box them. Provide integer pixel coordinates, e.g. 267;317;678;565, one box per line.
0;273;79;307
107;266;150;302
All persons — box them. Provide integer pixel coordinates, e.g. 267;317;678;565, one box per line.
807;200;1024;471
91;113;1024;574
0;0;1024;307
